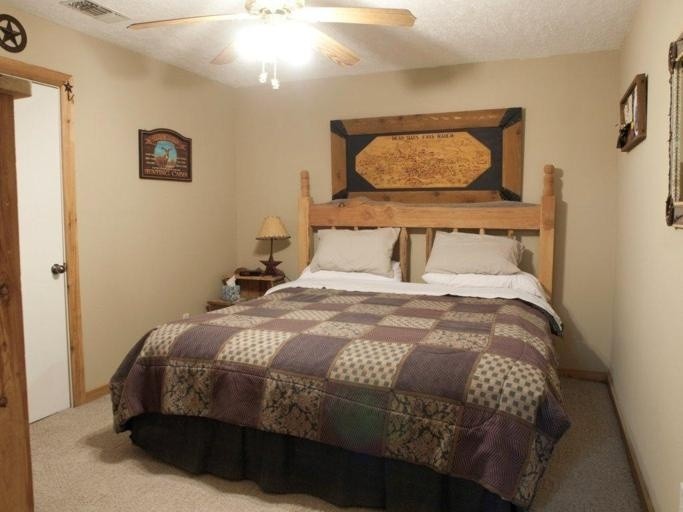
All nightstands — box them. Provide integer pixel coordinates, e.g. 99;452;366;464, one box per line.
224;274;284;299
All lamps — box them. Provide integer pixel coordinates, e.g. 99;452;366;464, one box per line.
256;216;291;276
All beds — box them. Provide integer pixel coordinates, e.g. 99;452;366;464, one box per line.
110;164;557;511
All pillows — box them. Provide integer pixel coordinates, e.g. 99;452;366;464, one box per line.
424;230;524;274
309;227;403;276
421;272;541;296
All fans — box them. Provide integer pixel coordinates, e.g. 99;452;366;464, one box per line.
123;0;416;66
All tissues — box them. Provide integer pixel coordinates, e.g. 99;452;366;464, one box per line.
222;276;240;301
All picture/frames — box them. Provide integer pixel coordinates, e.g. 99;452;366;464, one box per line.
620;73;647;153
138;127;192;182
331;107;523;201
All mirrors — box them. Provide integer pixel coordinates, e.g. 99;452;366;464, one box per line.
666;37;683;228
